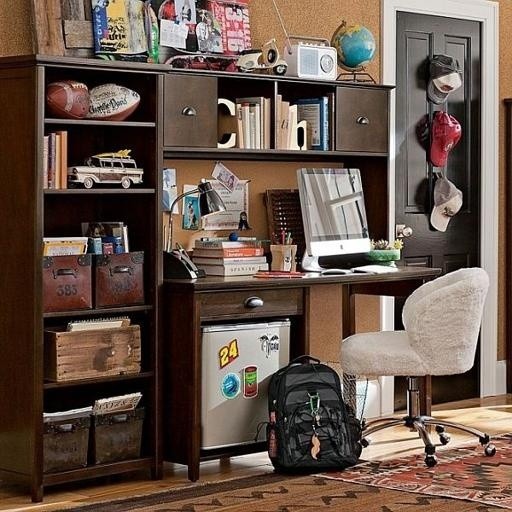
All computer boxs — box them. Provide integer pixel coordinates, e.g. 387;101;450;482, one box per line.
351;294;396;422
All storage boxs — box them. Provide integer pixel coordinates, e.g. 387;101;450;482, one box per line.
42;252;93;313
42;417;90;475
92;251;145;309
45;324;141;383
91;407;145;465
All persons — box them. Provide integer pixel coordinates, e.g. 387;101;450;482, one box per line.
224;175;234;191
187;208;196;229
216;173;222;180
88;224;102;237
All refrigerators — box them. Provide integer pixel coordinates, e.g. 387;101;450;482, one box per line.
198;320;290;450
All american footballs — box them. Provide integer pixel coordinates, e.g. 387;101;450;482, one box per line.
46;80;90;119
87;84;140;120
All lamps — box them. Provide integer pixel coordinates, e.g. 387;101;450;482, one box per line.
163;182;226;279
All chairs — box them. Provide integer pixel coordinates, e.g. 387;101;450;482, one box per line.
340;268;495;468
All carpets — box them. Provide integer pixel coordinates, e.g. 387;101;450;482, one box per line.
55;467;511;512
310;432;512;509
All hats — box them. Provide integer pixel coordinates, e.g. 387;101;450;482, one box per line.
429;110;463;168
430;168;463;233
427;54;465;105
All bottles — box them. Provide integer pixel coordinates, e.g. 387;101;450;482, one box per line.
266;399;277;458
244;366;258;398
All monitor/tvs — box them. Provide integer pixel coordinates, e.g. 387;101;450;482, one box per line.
296;168;373;271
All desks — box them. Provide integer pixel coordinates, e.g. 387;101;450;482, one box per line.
163;267;444;482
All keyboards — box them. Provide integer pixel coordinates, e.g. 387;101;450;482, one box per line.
352;265;398;274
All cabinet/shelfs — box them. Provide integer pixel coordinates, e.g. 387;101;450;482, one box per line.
1;54;396;503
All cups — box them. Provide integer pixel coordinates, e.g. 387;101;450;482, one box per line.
270;244;297;273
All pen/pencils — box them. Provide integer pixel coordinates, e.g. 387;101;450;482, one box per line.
272;231;295;245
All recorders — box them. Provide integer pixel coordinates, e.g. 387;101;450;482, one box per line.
285;36;337;81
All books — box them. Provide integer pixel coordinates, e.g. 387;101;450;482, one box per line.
94;390;141;415
192;257;267;263
67;320;123;331
43;405;92;424
101;242;114;254
195;239;262;249
104;236;123;254
83;316;131;327
193;263;271;277
41;129;68;191
90;236;102;254
233;92;334;150
192;247;265;257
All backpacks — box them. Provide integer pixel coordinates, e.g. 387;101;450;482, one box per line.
253;356;370;479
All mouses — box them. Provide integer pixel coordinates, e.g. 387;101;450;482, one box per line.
321;268;346;276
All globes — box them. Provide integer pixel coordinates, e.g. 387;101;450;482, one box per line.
330;19;376;85
